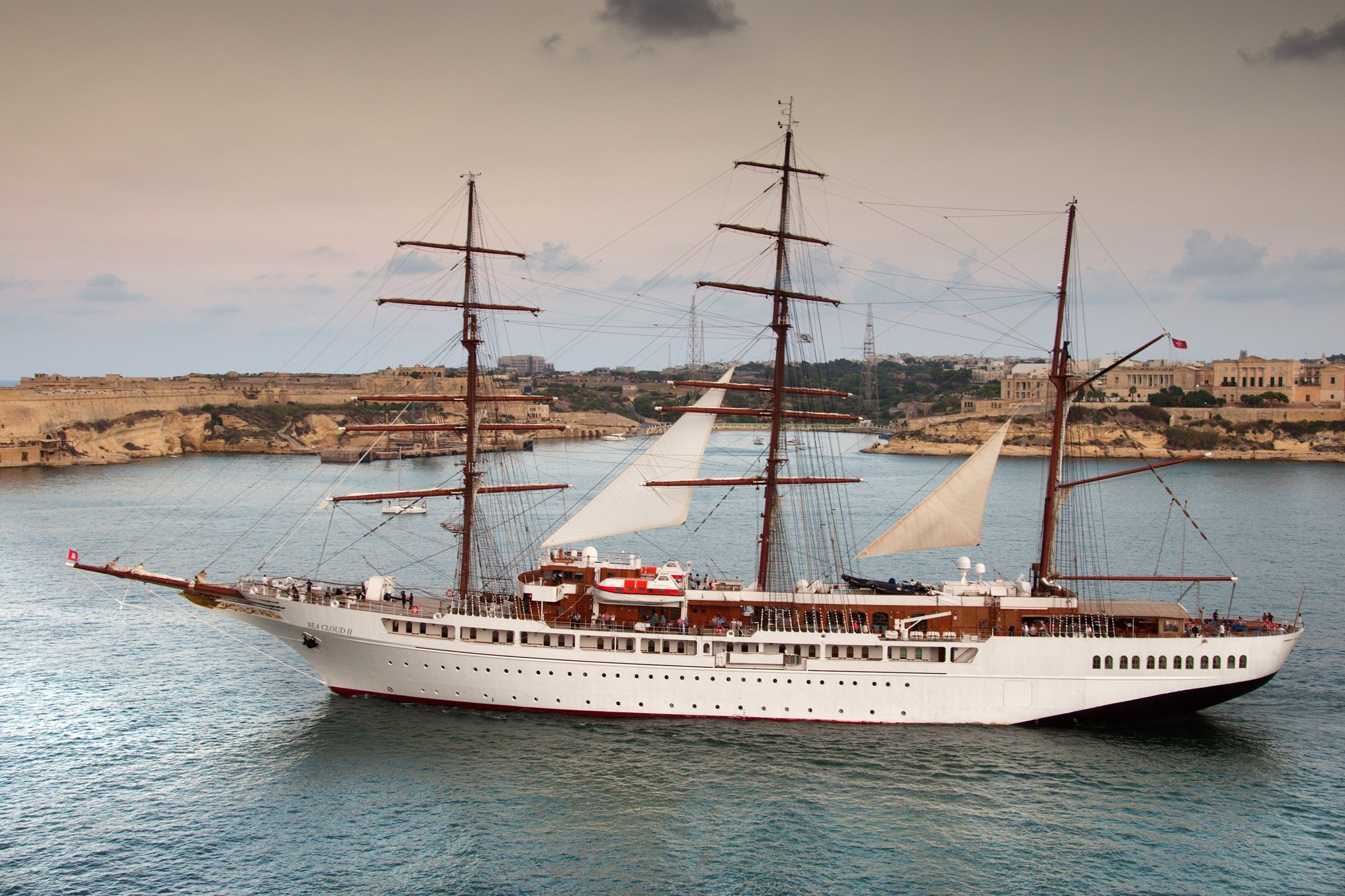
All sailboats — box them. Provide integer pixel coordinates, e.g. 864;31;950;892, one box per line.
62;95;1304;726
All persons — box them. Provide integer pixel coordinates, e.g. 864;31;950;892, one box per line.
538;560;1300;662
261;573;451;615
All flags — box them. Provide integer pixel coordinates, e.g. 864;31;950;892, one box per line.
68;549;78;561
1172;337;1187;349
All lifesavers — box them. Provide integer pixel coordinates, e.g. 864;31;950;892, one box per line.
411;605;419;614
1264;621;1272;625
1271;624;1279;628
445;589;454;597
1266;624;1273;629
